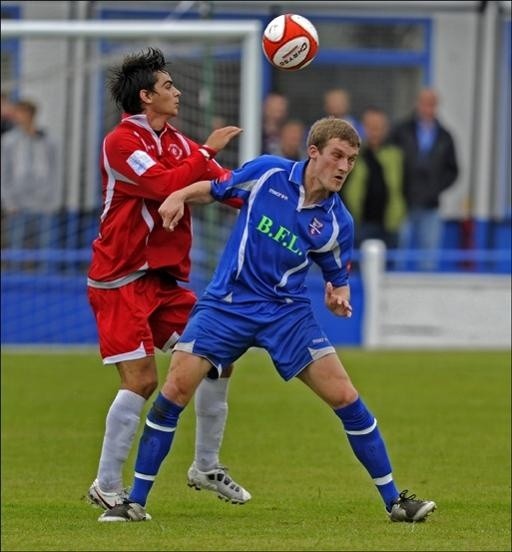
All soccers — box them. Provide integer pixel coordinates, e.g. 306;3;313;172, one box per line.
262;14;318;71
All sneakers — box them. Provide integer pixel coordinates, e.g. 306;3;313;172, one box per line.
384;490;436;521
184;461;252;505
86;478;153;523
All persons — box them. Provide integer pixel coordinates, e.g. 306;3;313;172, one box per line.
213;84;458;274
96;112;438;524
83;44;255;512
0;99;61;214
